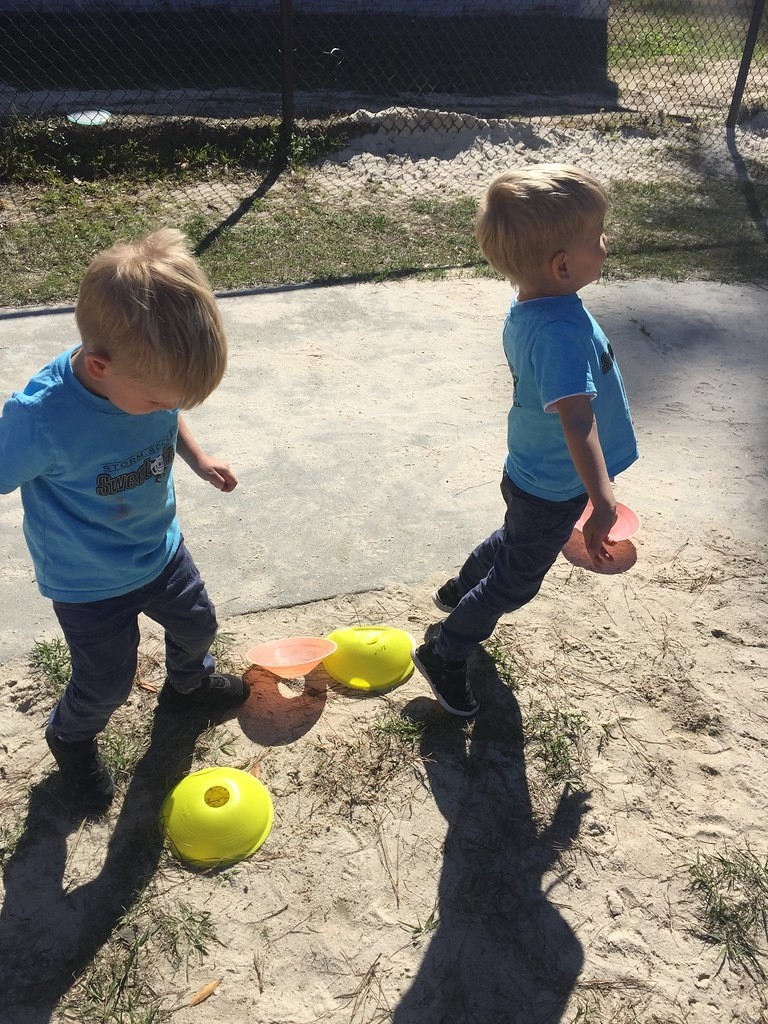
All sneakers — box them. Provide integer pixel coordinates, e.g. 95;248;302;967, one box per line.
45;724;116;810
431;578;461;613
410;636;480;716
156;674;251;712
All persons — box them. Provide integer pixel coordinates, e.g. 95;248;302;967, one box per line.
411;163;639;716
0;227;251;808
